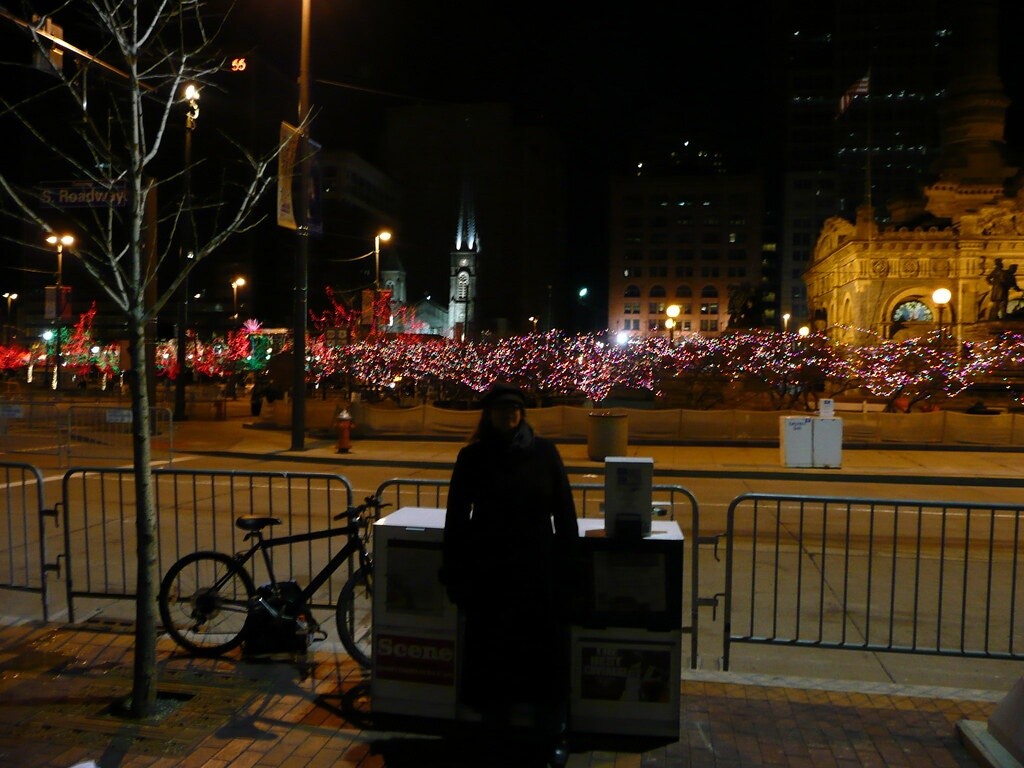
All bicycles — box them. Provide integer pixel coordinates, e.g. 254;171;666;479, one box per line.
156;493;397;672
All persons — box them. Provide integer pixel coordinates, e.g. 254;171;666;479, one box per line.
987;258;1012;320
438;382;580;768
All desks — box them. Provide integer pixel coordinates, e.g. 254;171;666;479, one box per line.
371;506;686;738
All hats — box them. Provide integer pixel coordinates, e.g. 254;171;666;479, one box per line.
483;388;525;408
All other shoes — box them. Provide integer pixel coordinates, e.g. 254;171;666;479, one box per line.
550;723;570;767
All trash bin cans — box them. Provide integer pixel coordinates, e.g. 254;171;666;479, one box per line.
570;514;685;741
373;504;467;719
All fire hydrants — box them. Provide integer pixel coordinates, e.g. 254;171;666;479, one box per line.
333;407;357;454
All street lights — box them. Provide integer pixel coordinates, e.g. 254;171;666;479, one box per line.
375;232;392;332
932;288;952;354
784;313;790;332
529;316;538;331
2;292;18;347
47;235;75;390
172;83;201;420
232;278;245;335
667;305;680;348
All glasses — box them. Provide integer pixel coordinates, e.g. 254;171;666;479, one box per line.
488;403;522;410
